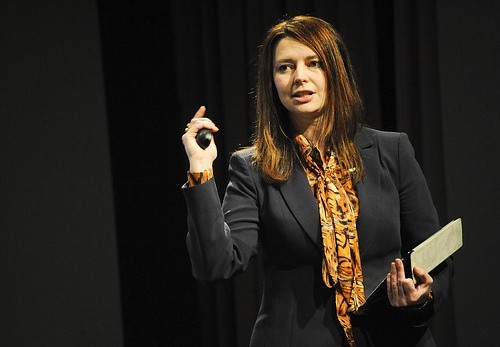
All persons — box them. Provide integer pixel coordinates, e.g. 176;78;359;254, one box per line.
181;14;449;347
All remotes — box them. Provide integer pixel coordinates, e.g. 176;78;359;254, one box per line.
196;128;211;149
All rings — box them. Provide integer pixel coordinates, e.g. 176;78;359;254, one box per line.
185;123;191;132
